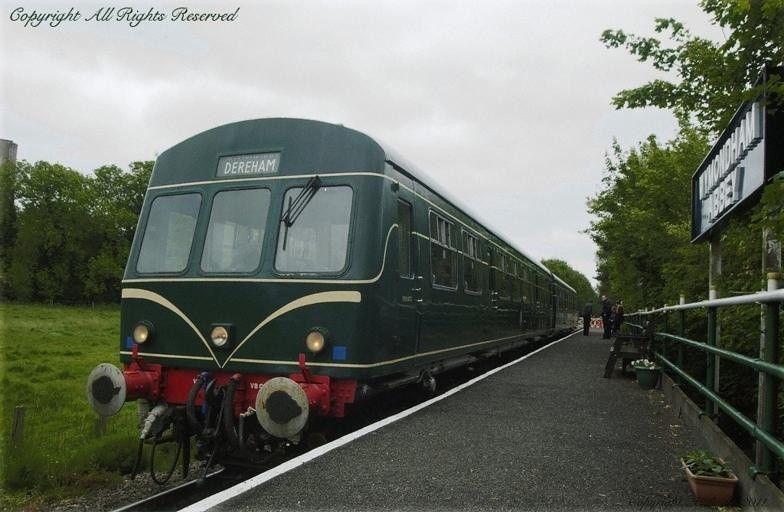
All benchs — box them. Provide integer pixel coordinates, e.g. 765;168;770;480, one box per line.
603;336;642;377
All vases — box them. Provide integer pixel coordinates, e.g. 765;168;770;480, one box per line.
635;368;660;391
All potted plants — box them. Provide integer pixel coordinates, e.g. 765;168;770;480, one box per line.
674;445;739;507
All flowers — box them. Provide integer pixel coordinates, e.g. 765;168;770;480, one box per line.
630;357;658;371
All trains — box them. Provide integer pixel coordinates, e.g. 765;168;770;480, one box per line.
84;115;580;489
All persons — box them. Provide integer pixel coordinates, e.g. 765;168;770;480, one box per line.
581;298;593;336
600;294;612;340
610;299;624;338
